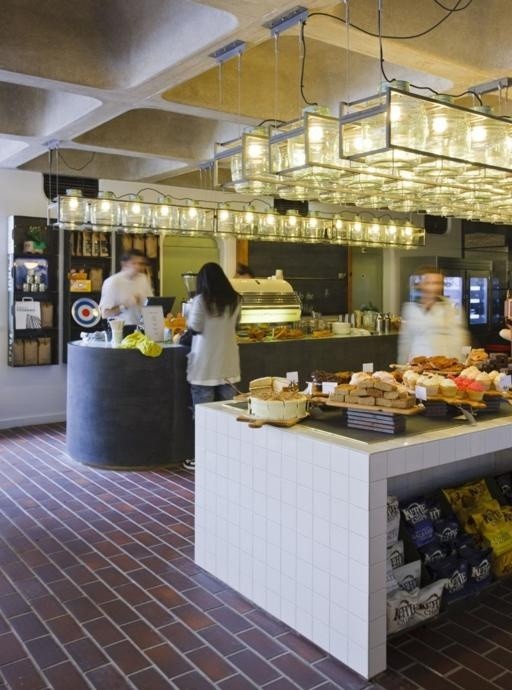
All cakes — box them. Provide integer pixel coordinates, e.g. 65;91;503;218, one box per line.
402;366;509;400
248;391;306;420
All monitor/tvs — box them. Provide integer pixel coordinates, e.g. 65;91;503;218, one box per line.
141;297;176;318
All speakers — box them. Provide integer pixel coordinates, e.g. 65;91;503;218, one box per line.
424;215;448;234
274;198;308;217
43;173;99;202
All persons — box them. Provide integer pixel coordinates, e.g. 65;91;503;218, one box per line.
99;248;157;341
394;268;472;372
171;262;243;471
236;262;256;279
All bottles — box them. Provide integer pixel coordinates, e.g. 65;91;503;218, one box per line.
22;273;46;293
375;317;391;333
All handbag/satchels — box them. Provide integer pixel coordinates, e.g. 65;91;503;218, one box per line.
14;296;41;330
42;299;54;329
13;332;52;366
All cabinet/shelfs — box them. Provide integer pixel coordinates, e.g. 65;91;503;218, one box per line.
6;214;165;368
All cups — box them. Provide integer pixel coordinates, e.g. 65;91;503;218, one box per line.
109;319;125;345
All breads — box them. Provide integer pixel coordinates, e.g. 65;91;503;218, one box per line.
329;377;414;408
407;355;467;374
468;349;508;371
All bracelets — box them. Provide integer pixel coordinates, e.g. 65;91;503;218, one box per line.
118;304;127;314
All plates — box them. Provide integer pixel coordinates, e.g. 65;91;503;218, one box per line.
331;322;352;335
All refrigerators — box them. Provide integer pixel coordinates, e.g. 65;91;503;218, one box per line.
398;254;494;351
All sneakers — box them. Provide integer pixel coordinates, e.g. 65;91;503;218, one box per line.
181;454;196;471
